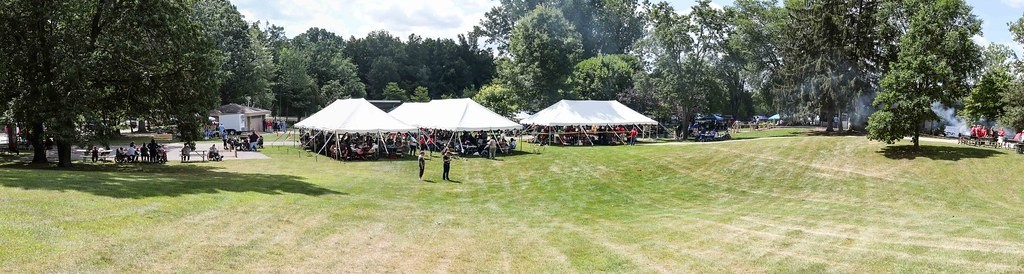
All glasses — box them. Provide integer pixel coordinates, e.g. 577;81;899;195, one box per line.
213;144;215;146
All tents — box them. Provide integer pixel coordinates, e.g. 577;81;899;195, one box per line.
768;114;789;121
690;108;733;129
752;112;767;121
293;95;659;136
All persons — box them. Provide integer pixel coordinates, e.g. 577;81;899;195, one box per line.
834;115;838;128
208;143;224;162
114;139;169;163
263;116;287;134
525;119;657;148
201;122;225;138
92;145;98;161
181;142;191;161
222;128;264;157
971;122;1024;149
418;151;426;179
442;151;452;181
747;115;821;130
297;126;521;161
156;126;175;135
671;116;743;138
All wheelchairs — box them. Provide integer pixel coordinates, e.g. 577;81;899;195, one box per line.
114;149;128;163
207;152;223;161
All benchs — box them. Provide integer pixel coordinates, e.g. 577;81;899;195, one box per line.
179;150;208;162
153;133;173;144
80;152;116;162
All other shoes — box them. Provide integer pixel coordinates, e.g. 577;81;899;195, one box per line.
419;178;422;181
443;178;445;180
631;143;632;146
633;143;634;145
184;160;186;162
224;147;229;150
187;160;190;162
446;177;449;180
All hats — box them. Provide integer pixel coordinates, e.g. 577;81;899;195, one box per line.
491;137;495;139
633;127;636;130
510;138;514;140
420;151;425;154
446;151;452;154
